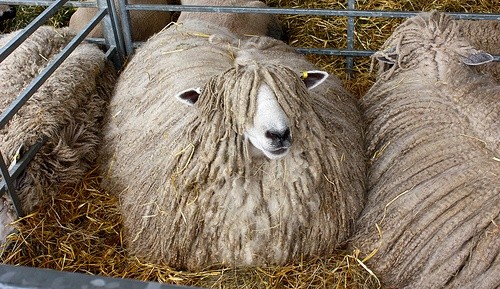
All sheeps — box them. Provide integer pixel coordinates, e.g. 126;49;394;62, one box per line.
0;0;500;289
